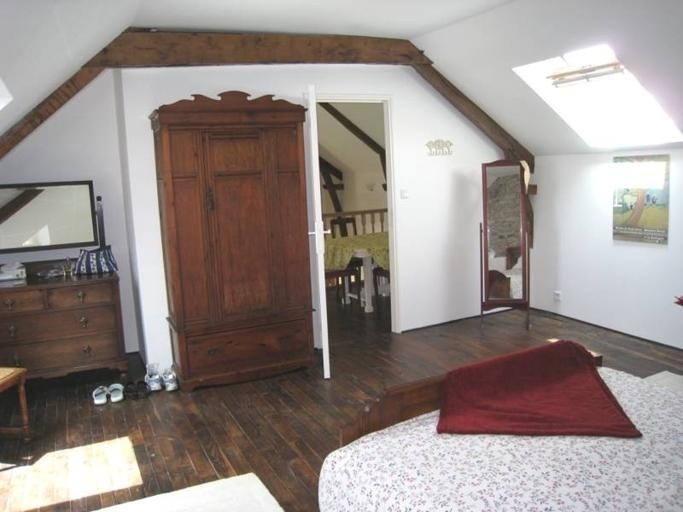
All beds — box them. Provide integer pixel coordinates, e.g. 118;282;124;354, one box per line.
316;336;683;512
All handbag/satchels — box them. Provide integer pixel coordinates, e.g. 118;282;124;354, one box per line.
74;245;118;275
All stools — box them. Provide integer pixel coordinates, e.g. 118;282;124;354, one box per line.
0;365;31;439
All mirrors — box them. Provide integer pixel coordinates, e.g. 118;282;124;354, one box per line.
1;178;97;252
480;159;532;331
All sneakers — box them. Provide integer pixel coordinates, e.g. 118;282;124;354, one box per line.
144;362;162;391
161;368;178;392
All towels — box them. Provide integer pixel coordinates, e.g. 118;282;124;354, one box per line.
433;338;642;441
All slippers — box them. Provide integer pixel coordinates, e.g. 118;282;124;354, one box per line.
107;383;124;402
137;381;148;399
92;385;108;404
125;381;137;400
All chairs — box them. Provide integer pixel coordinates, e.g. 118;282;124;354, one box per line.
323;216;390;313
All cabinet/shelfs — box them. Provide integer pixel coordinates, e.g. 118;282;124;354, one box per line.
0;267;127;390
147;90;317;391
